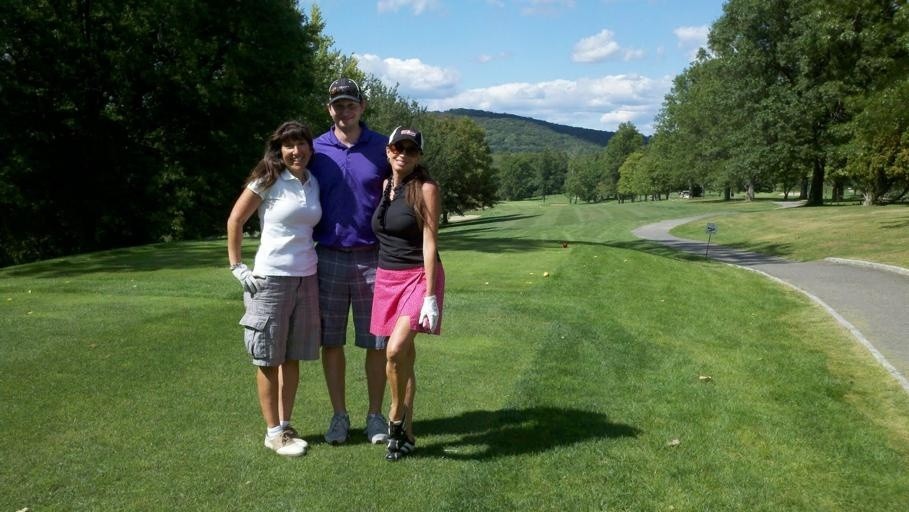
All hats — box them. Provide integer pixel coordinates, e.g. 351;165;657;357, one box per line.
386;125;424;152
326;78;362;105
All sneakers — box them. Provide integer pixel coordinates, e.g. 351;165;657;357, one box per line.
384;437;416;462
386;404;407;452
364;412;389;447
265;428;307;456
280;423;308;448
323;411;352;446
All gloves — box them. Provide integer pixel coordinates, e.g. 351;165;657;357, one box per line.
229;260;268;295
418;295;439;333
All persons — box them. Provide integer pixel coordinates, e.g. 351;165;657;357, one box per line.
306;77;389;448
367;124;446;462
225;120;322;456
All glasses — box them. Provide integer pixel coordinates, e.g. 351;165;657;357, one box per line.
388;143;421;158
